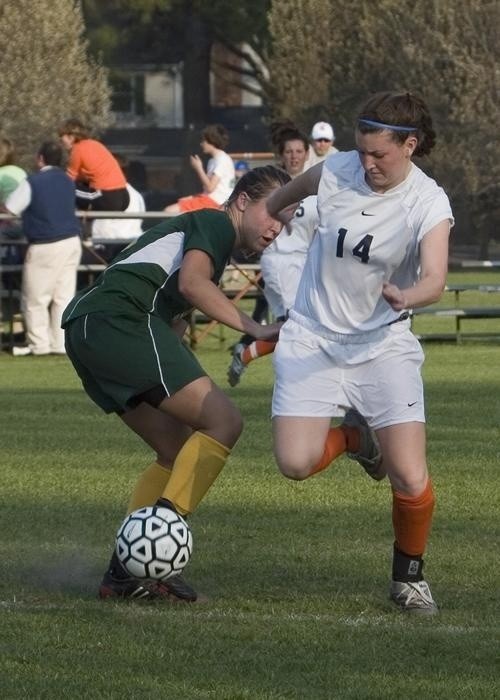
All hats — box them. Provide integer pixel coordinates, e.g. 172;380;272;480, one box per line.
309;120;337;143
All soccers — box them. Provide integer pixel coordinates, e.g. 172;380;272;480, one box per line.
115;506;194;580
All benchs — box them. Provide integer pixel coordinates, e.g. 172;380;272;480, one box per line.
0;210;265;352
410;259;500;343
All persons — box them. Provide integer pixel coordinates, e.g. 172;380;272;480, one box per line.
1;117;146;356
159;122;339;358
59;164;298;602
265;90;454;623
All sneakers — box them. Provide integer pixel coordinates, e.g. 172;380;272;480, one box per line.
13;342;67;358
97;570;180;604
156;572;200;603
339;406;390;483
225;340;247;388
387;577;440;624
